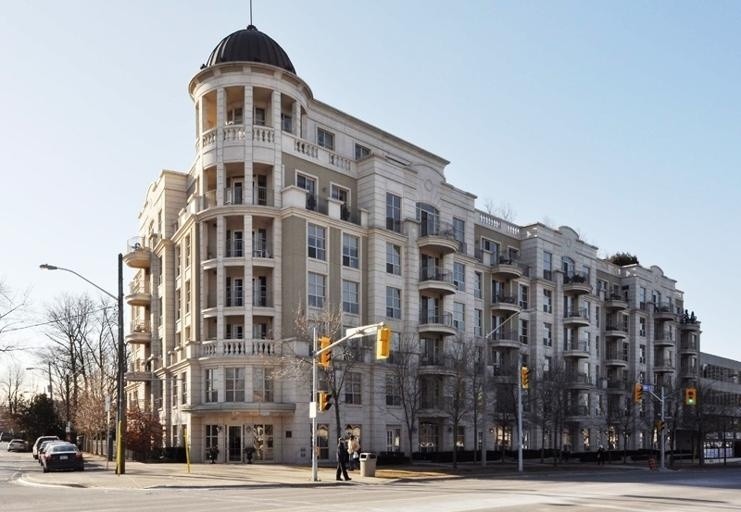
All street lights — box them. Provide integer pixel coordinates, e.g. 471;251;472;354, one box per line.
699;374;739;466
39;264;125;474
26;368;71;441
481;308;536;469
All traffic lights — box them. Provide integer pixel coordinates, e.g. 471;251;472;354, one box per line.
320;337;331;368
635;383;642;402
686;388;696;404
319;393;333;412
521;367;530;389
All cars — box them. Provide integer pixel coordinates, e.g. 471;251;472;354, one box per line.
32;435;84;473
0;432;28;452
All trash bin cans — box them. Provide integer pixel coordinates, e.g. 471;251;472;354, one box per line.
359;453;377;477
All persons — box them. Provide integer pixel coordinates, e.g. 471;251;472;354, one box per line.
346;433;362;471
597;448;606;466
336;436;352;481
593;446;601;464
